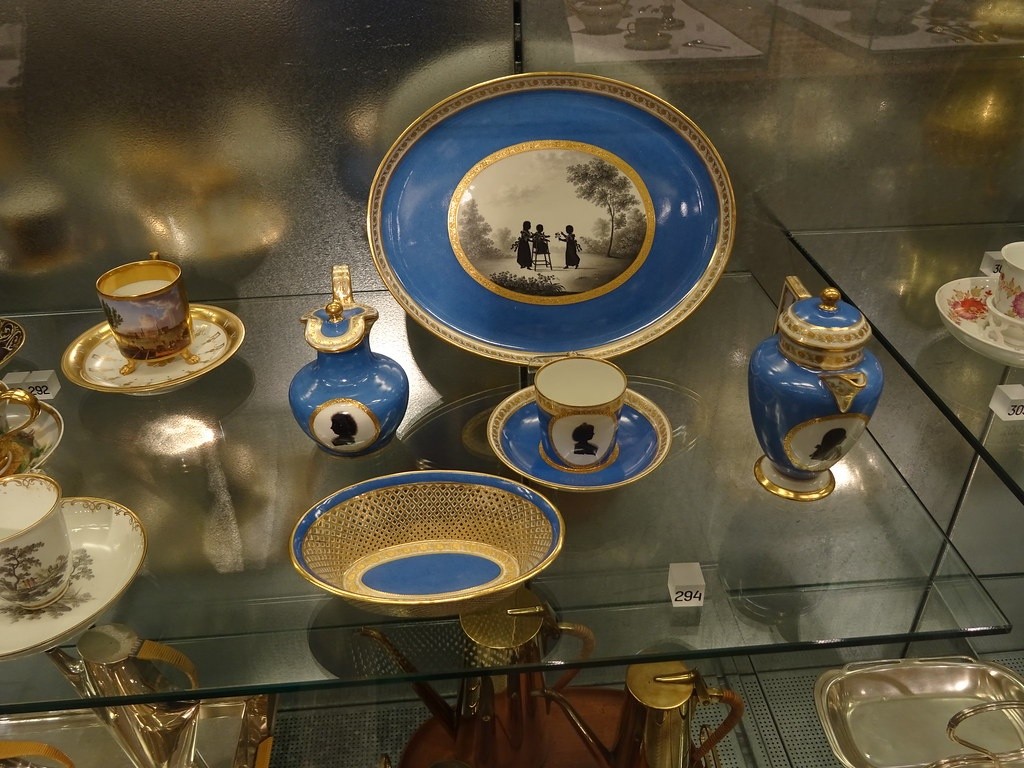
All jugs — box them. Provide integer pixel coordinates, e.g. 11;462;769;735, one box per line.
45;625;199;768
358;592;592;768
541;647;742;768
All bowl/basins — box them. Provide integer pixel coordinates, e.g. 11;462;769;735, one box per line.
986;296;1024;347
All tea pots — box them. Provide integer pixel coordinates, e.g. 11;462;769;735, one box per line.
290;262;407;461
746;275;885;502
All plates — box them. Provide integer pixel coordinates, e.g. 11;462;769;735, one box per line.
624;33;671;49
935;277;1024;370
366;70;735;367
60;303;245;395
0;317;26;366
0;401;63;475
0;496;146;659
487;388;672;493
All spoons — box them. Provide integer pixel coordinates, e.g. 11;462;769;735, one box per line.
684;43;722;52
640;5;651;13
927;21;999;44
693;39;731;49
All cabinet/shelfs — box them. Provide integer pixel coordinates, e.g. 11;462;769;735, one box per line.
0;221;1024;768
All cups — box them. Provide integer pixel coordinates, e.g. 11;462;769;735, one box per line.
0;380;40;474
626;17;660;39
534;352;628;469
93;251;191;364
994;242;1024;322
0;474;74;610
